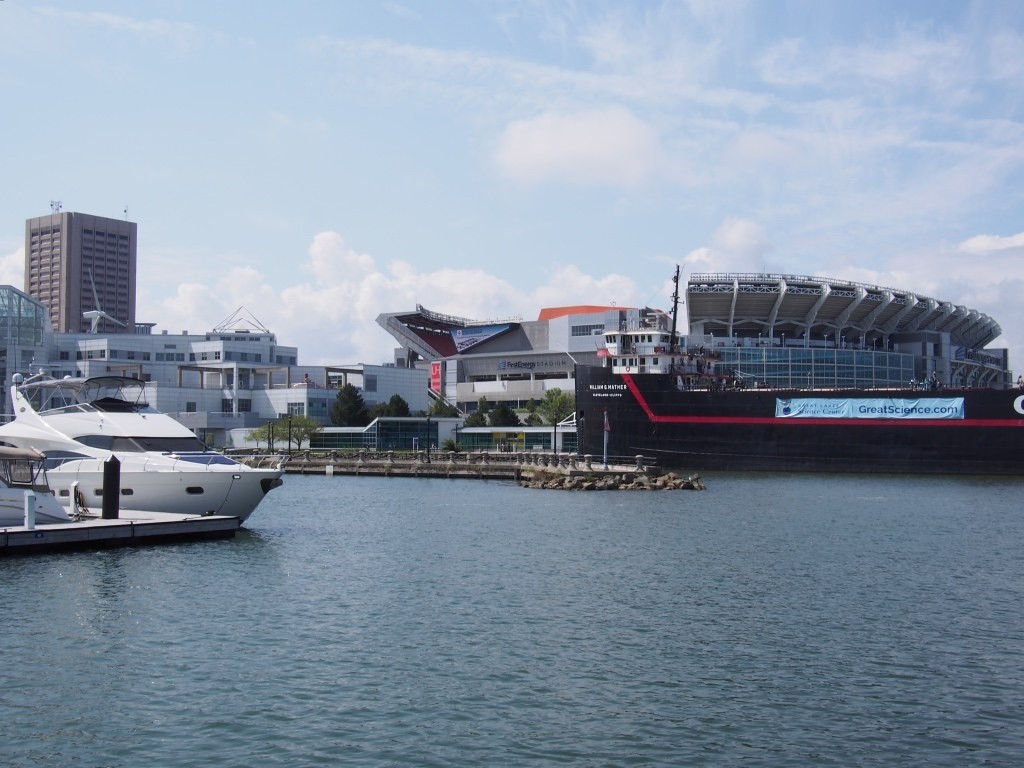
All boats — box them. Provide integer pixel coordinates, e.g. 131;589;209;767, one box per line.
0;366;291;526
0;444;74;525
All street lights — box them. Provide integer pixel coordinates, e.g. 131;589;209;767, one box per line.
553;410;558;453
288;415;292;461
267;421;274;455
455;423;458;445
427;413;431;464
